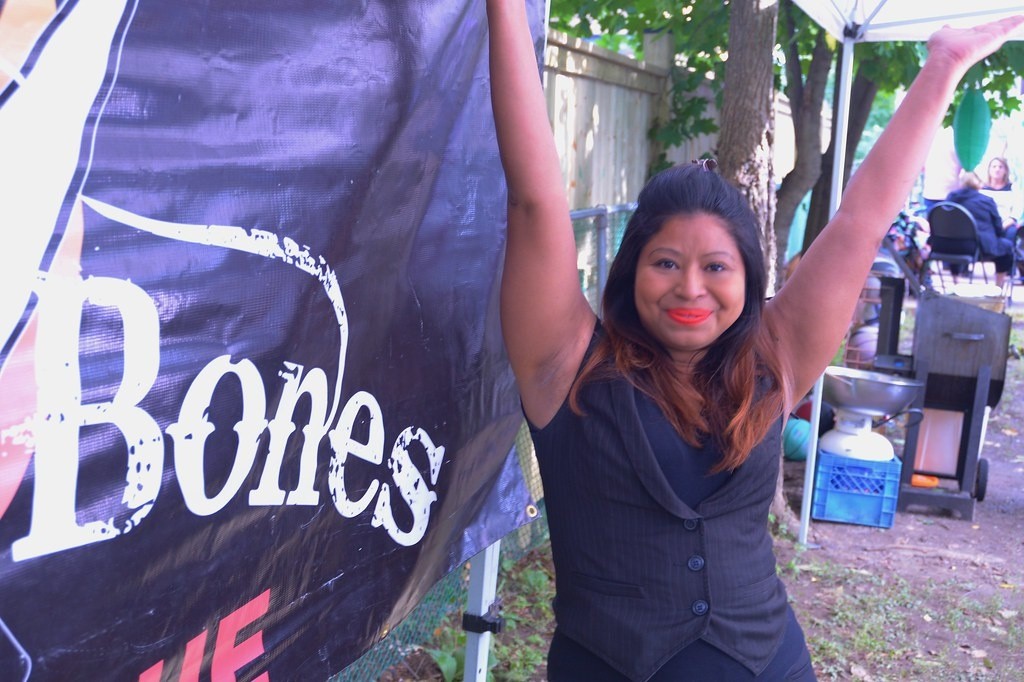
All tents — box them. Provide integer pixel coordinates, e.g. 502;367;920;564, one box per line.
760;0;1024;547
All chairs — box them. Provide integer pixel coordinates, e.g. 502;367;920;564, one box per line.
926;201;1024;306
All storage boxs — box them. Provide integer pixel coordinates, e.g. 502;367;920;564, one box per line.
810;437;902;529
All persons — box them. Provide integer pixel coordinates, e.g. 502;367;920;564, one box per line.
924;124;1022;288
485;0;1024;682
